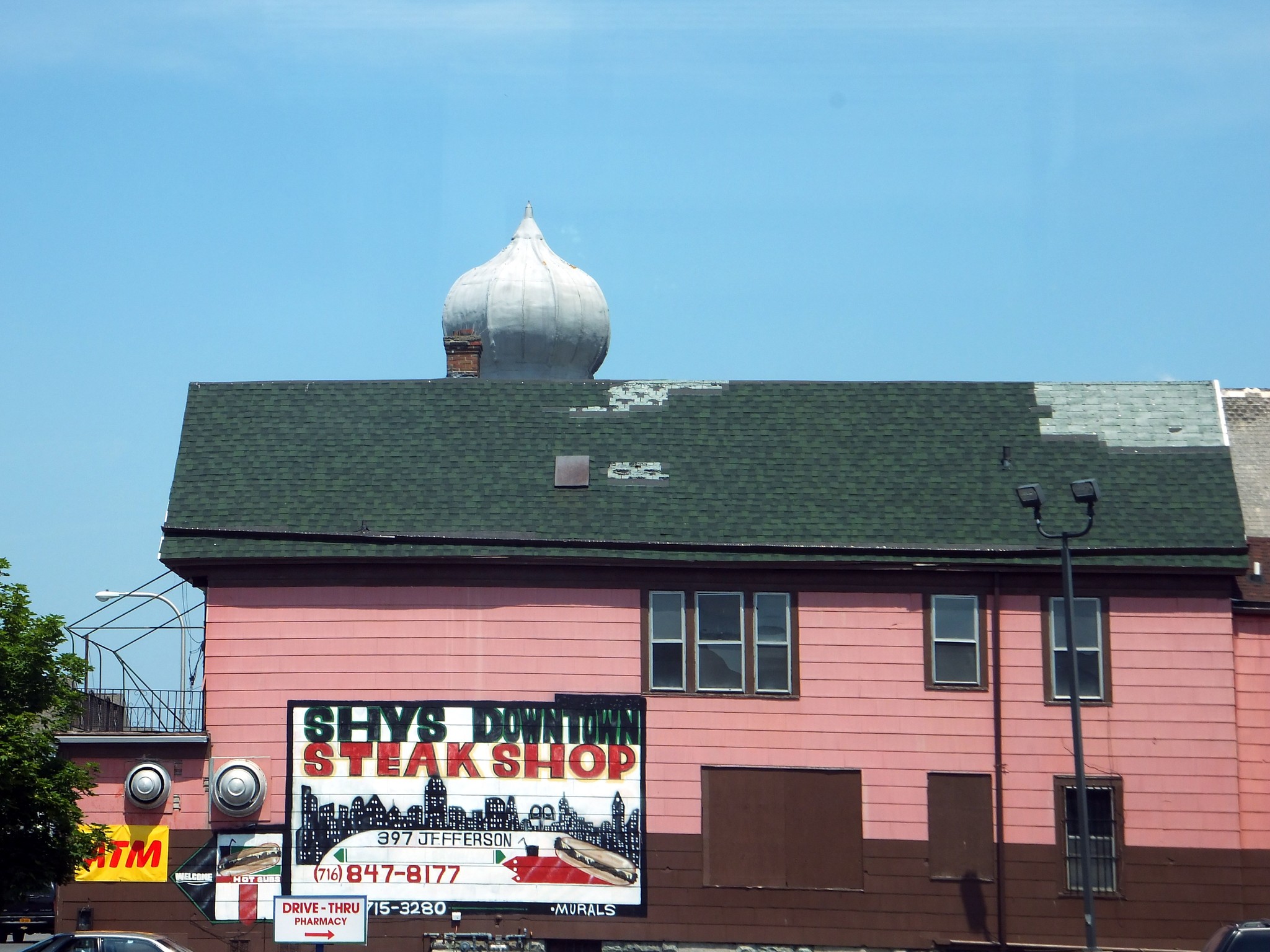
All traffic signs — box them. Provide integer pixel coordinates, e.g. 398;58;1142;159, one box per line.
272;895;368;947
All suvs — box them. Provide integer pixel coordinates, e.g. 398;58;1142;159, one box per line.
0;882;56;942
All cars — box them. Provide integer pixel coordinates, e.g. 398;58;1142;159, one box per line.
19;931;194;952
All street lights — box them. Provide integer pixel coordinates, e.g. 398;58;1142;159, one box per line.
94;589;185;732
1016;478;1102;951
